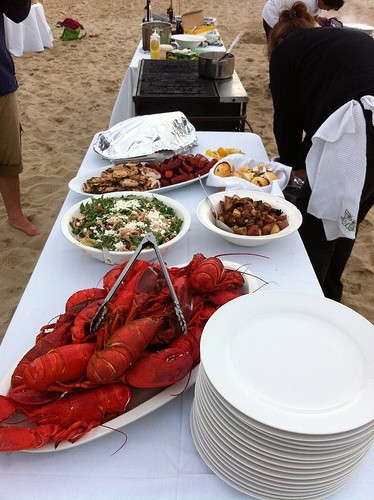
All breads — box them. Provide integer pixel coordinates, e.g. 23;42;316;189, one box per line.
206;146;241;160
214;161;277;187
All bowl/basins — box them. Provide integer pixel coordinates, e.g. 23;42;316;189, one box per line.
342;23;374;36
171;35;207;48
197;190;303;246
59;190;191;265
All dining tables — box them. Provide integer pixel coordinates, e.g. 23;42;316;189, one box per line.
0;131;374;499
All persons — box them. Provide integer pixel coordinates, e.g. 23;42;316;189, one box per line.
269;1;374;302
262;0;344;38
0;0;36;234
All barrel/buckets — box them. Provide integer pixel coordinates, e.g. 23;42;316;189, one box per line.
141;22;173;49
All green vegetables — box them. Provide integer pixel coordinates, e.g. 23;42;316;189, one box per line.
70;193;184;252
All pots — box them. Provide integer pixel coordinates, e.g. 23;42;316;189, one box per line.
198;52;236;79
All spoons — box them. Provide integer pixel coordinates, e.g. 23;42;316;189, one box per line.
198;174;235;234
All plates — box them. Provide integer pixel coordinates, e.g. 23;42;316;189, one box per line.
0;261;257;453
92;139;172;162
190;288;374;500
68;154;217;197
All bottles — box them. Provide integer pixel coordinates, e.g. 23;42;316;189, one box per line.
150;29;160;53
167;0;173;21
176;18;183;34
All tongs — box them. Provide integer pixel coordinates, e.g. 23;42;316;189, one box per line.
89;232;187;337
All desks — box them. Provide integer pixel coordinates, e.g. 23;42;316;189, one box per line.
108;37;226;129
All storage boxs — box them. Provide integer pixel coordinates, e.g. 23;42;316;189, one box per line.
182;9;214;34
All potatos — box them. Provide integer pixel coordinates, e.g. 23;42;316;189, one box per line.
219;196;288;236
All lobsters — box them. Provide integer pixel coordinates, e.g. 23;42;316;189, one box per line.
0;252;246;453
